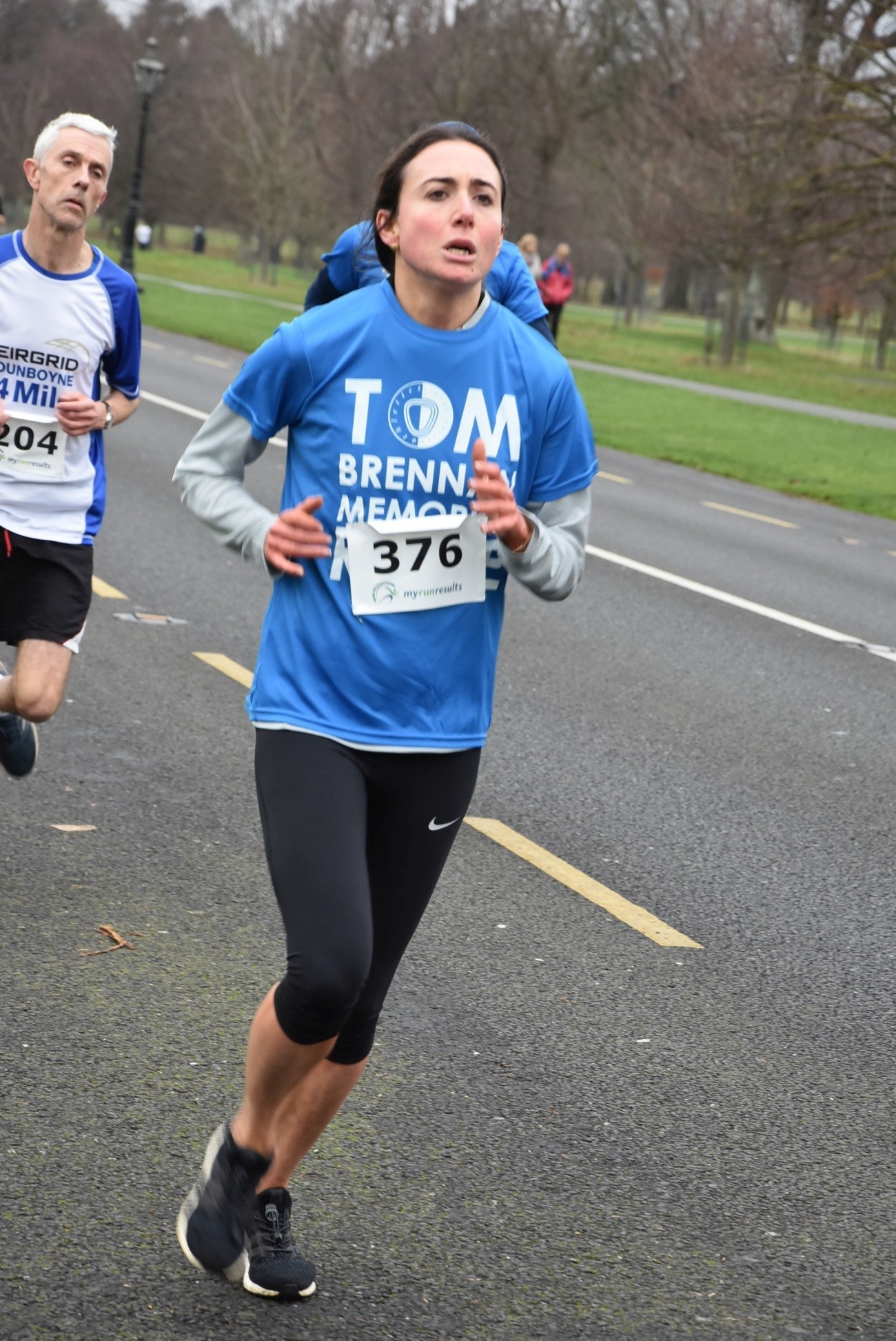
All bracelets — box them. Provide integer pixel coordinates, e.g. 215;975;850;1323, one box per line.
515;524;533;552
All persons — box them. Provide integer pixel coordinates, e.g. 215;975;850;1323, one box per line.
537;242;574;340
517;233;541;280
303;221;555;345
136;220;152;249
0;113;142;779
174;120;596;1301
193;226;204;251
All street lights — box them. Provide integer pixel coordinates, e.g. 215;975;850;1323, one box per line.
119;36;166;295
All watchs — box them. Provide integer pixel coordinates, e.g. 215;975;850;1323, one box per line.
101;401;113;429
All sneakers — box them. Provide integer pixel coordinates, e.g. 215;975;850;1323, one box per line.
0;661;40;778
223;1186;317;1298
175;1121;276;1275
